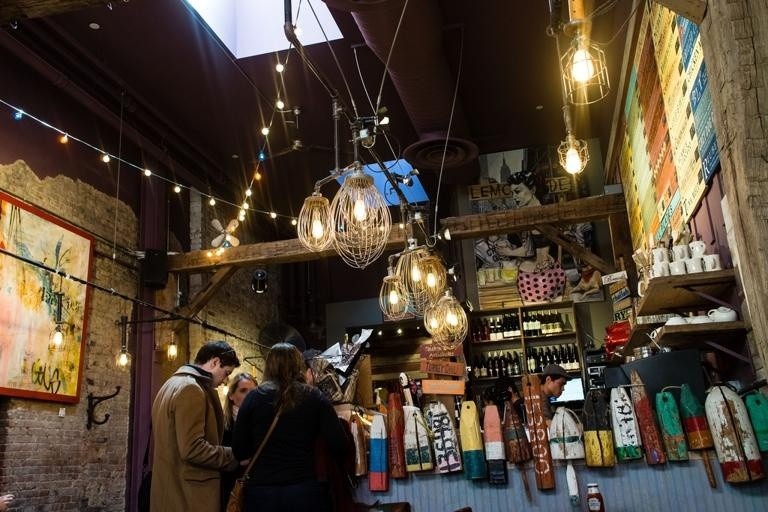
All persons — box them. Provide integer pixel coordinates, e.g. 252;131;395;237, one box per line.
479;388;503;427
493;378;524;421
540;365;571;426
221;374;257;448
492;171;558;273
1;493;17;510
233;343;355;511
150;341;234;511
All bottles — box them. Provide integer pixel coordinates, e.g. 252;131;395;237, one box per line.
586;482;605;511
470;341;581;380
470;307;565;341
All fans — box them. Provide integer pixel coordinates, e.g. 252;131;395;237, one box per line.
210;218;239;248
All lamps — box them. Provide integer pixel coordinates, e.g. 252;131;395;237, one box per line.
248;262;269;298
559;22;612;105
557;109;590;175
268;0;469;354
111;308;183;371
48;274;70;353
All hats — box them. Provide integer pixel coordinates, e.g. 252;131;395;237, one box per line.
543;364;573;381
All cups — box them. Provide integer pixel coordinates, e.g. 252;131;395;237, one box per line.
651;239;721;278
625;307;736;364
474;264;501;289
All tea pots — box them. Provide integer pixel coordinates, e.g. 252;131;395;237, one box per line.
501;259;519;282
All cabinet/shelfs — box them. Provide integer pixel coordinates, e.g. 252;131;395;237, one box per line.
345;300;591;418
620;265;756;379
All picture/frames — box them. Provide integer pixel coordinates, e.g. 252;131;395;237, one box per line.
0;191;96;404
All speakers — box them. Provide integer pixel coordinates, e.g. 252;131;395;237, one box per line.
143;250;169;290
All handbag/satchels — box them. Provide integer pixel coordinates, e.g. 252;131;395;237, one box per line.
226;478;248;512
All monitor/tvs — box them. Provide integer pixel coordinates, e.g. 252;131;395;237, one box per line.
548;375;586;406
